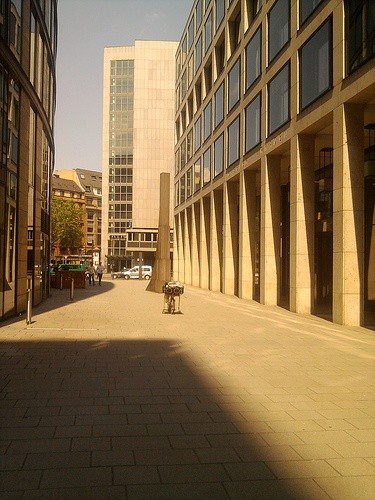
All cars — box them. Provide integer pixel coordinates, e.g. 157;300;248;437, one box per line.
111;266;132;279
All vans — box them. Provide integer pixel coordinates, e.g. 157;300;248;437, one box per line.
50;264;84;277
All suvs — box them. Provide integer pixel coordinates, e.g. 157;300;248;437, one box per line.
124;265;152;279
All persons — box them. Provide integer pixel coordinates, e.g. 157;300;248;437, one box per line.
96;262;104;286
88;263;96;286
